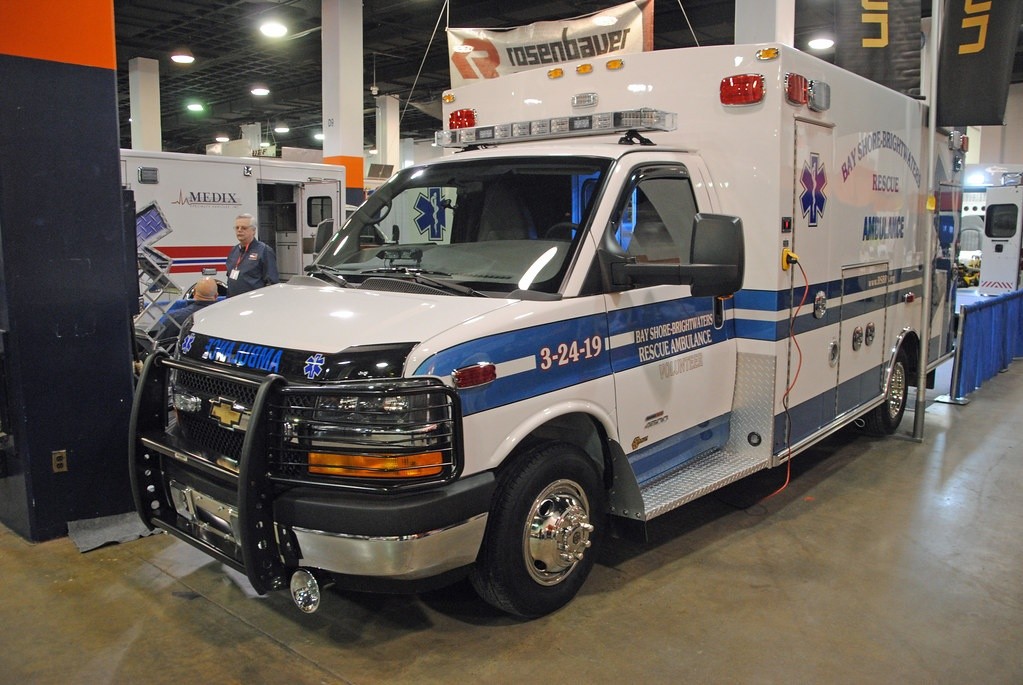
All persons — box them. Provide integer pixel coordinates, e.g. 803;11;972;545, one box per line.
227;212;280;299
134;276;219;378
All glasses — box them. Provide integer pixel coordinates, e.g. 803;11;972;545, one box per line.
234;225;252;231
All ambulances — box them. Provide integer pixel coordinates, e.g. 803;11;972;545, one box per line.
128;42;971;621
120;149;346;348
